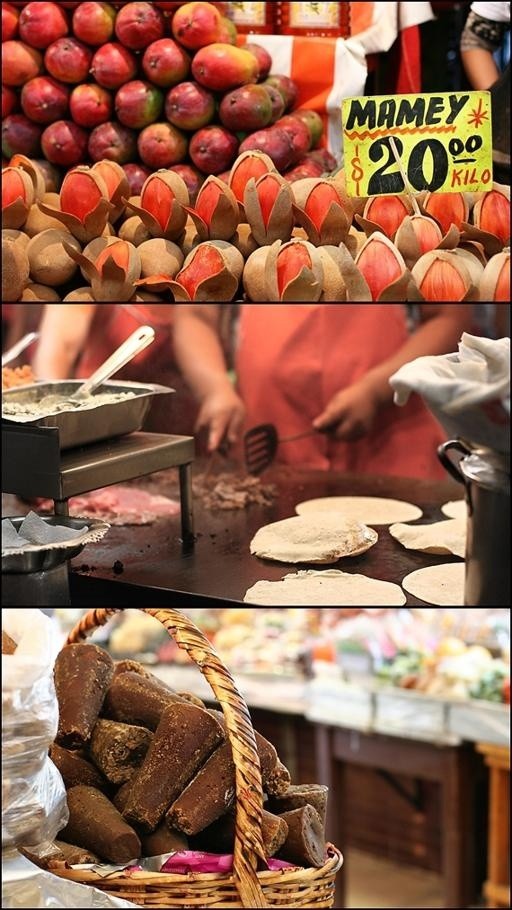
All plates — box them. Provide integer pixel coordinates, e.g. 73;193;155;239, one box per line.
1;512;112;575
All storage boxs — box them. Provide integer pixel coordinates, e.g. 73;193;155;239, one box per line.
239;672;305;715
446;700;512;745
372;696;446;742
143;668;229;700
305;681;377;735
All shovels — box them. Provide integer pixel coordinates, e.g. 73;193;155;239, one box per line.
243;421;340;476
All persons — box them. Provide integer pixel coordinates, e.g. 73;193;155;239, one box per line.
459;0;510;187
2;304;479;484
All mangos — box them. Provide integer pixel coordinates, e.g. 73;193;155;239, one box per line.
1;0;510;301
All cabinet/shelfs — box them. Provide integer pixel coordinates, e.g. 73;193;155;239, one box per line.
314;729;483;909
200;699;510;909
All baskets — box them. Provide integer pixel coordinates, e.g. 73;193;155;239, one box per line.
21;607;344;910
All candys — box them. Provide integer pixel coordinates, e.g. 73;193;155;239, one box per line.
52;639;332;873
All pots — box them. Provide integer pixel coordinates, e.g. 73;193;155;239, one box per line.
429;441;512;609
